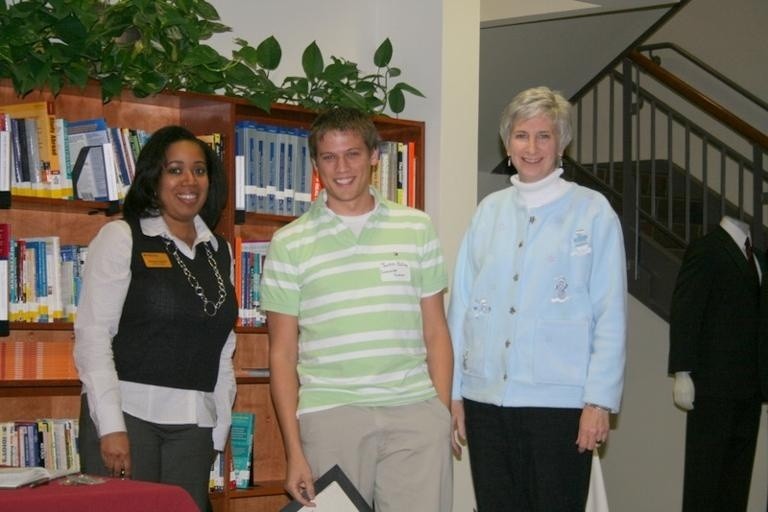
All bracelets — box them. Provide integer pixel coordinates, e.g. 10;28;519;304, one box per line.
584;402;609;413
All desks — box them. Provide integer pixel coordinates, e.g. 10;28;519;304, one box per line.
0;471;200;512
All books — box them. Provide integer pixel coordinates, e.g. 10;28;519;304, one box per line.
235;236;270;328
2;418;82;489
1;102;222;206
0;224;90;325
235;120;416;217
208;410;253;499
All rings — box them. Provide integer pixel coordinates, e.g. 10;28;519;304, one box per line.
595;439;602;444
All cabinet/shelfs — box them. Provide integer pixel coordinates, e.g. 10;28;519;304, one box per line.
0;74;425;512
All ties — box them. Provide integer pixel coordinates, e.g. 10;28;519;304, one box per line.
744;237;760;286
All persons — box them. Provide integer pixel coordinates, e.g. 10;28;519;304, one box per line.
255;106;455;511
667;216;768;512
72;125;237;512
447;87;626;511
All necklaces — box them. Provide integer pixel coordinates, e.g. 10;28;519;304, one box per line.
159;230;227;317
